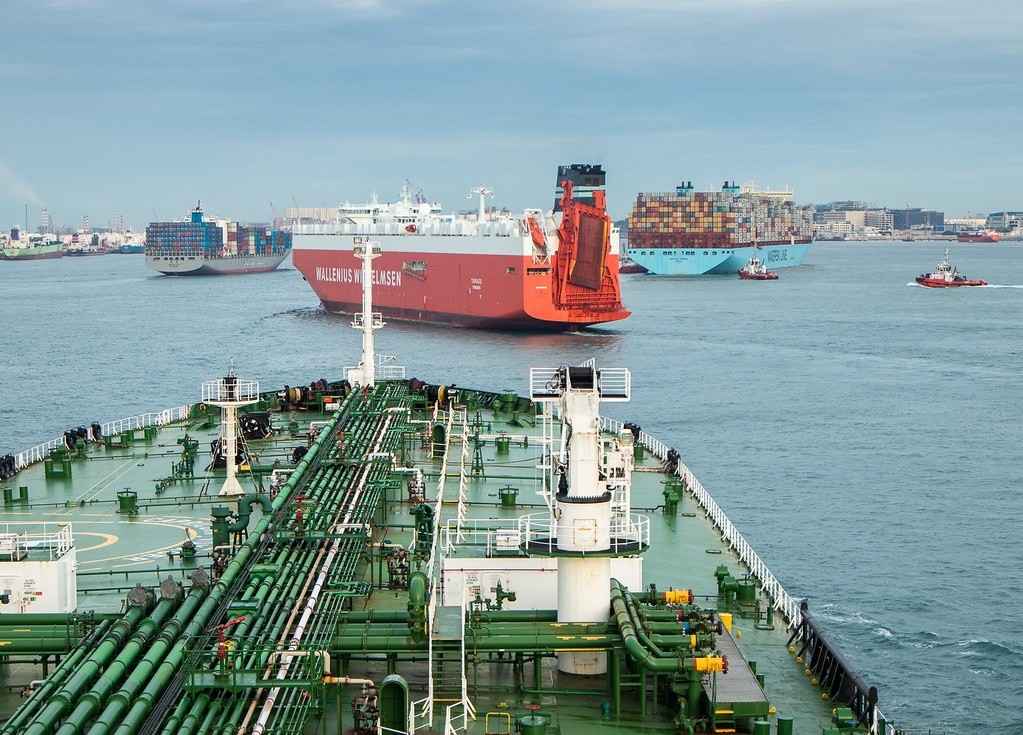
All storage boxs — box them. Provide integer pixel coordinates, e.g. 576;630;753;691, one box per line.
627;190;816;249
145;221;293;260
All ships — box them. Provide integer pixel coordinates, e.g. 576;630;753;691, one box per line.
145;248;293;276
626;241;816;277
288;163;634;335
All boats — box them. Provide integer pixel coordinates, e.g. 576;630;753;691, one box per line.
956;229;1001;242
915;248;988;288
71;247;105;257
120;243;146;254
619;264;648;274
2;242;62;260
738;239;778;280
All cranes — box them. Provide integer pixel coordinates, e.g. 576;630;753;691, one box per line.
269;196;328;225
